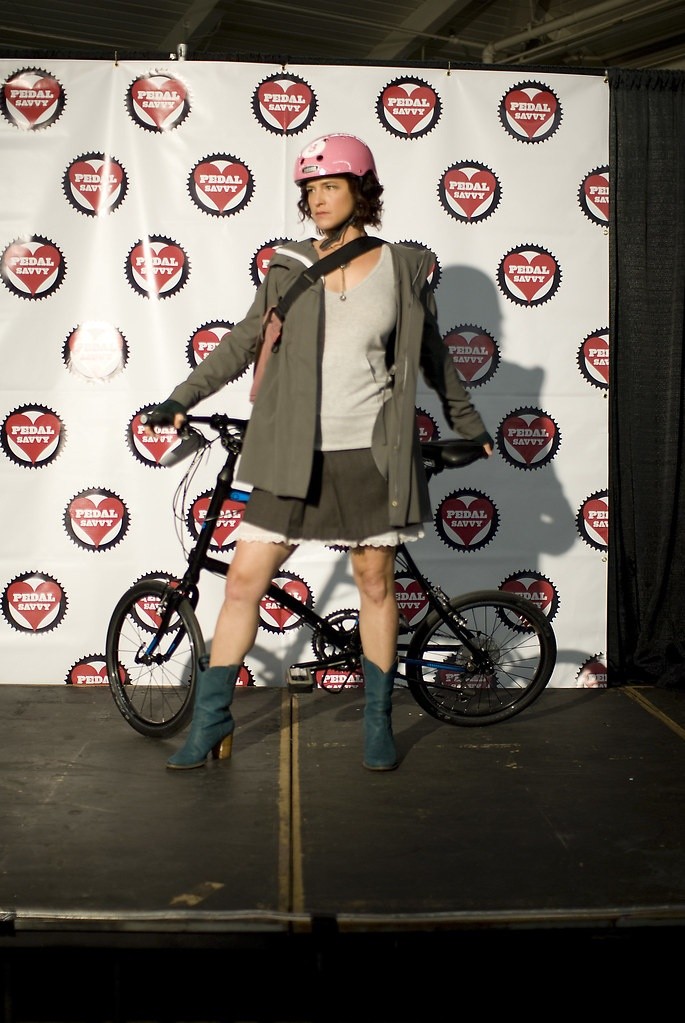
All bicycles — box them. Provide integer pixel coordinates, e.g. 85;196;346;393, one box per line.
105;411;560;738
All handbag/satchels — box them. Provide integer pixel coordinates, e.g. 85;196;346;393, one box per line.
247;306;286;403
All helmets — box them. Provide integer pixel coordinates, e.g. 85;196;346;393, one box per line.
296;134;375;186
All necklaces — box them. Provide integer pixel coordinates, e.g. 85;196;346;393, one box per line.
324;236;348;300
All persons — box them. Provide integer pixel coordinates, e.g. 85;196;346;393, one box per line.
149;131;496;769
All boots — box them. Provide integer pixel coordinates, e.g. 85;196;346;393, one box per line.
165;662;245;770
360;655;402;770
